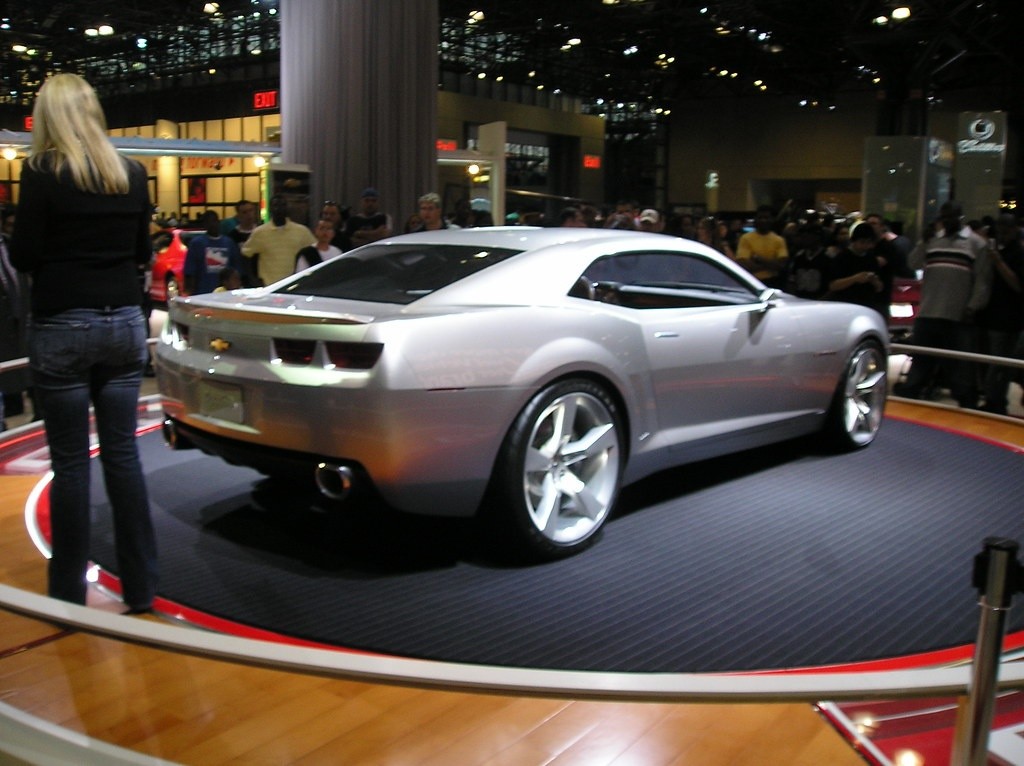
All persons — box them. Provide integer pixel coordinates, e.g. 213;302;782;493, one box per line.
7;73;160;615
137;191;1024;417
0;199;46;433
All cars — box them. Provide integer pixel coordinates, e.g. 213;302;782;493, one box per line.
880;230;924;331
150;225;889;566
145;229;233;310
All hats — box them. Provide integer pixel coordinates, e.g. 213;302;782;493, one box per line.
469;198;492;213
640;209;659;223
850;224;874;240
361;188;378;197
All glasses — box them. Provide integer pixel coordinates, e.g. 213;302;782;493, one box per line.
324;200;337;205
